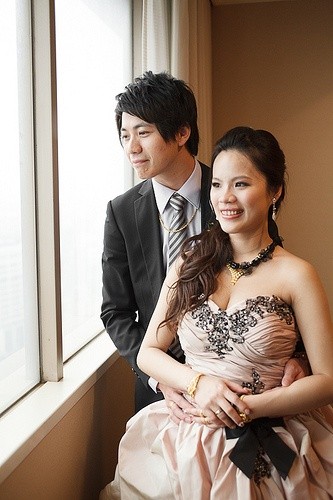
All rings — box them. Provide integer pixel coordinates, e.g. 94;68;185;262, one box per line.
205;418;211;424
200;409;207;419
215;409;222;415
167;401;175;408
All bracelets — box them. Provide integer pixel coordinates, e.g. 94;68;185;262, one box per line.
186;372;204;397
239;393;251;423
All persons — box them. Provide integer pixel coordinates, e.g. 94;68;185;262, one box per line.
101;126;333;500
101;70;312;416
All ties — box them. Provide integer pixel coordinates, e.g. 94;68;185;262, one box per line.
168;193;189;358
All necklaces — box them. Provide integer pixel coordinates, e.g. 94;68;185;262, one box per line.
159;209;197;232
224;240;277;286
